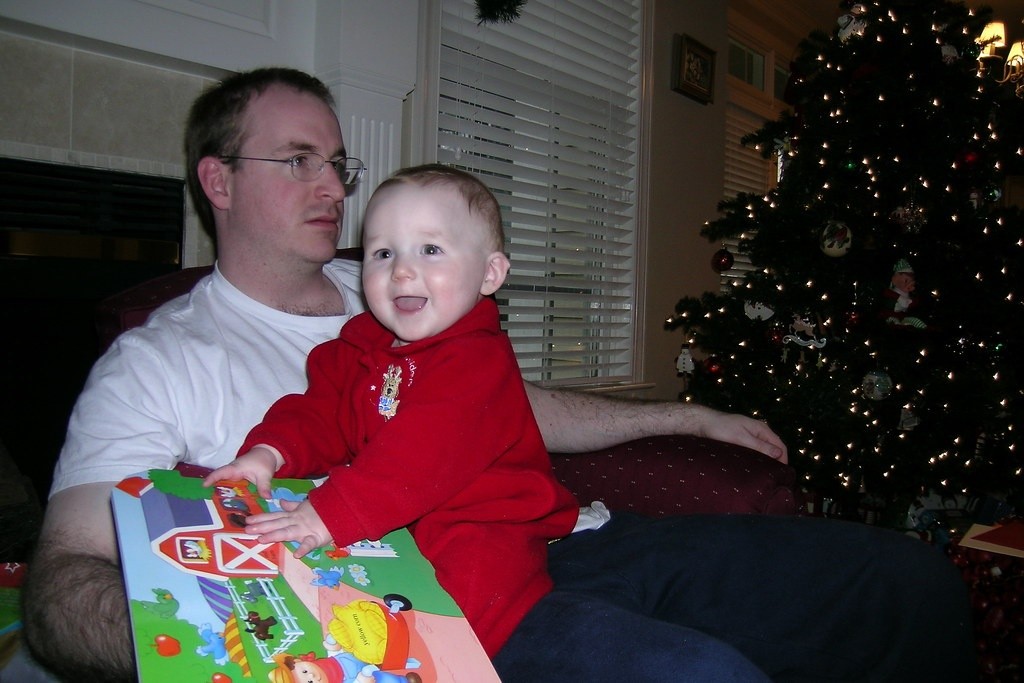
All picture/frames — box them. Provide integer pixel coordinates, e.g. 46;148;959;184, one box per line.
671;33;717;104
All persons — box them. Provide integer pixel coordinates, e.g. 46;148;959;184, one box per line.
201;161;579;662
20;65;971;682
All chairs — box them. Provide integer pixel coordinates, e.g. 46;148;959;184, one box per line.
124;266;811;520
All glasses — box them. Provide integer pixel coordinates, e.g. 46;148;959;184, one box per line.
219;153;367;186
